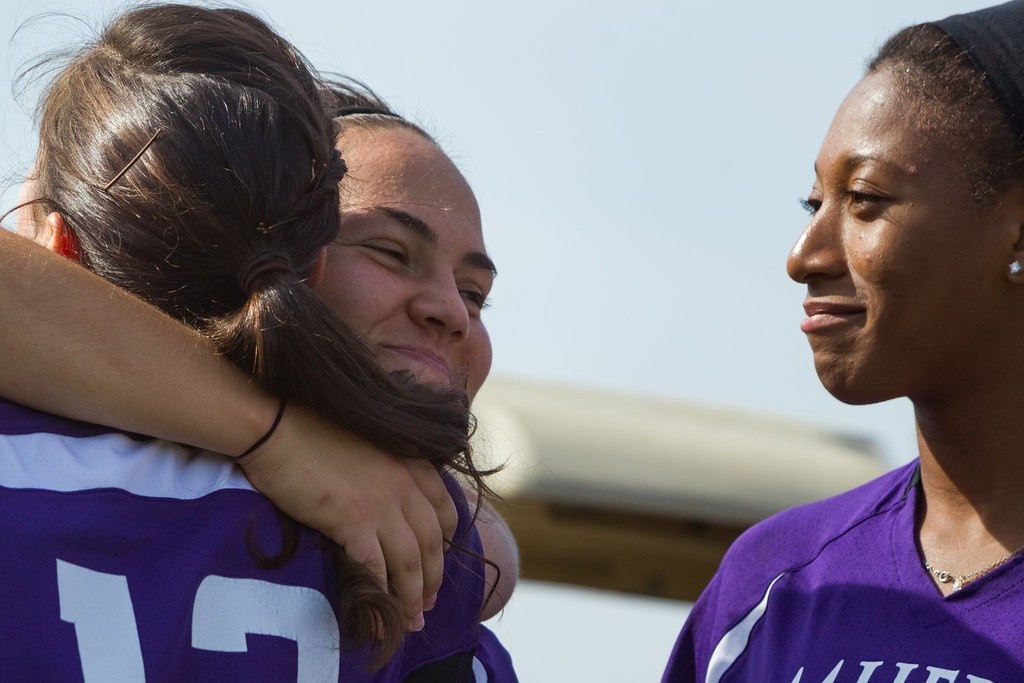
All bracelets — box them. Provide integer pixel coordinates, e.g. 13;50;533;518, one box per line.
225;402;287;462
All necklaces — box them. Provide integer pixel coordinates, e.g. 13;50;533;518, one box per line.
922;546;1024;593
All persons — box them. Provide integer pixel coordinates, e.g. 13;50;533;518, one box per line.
0;2;520;683
657;0;1024;683
